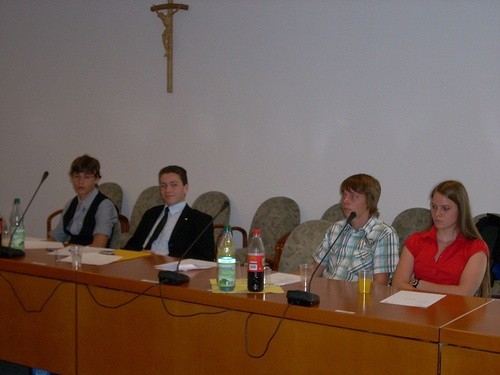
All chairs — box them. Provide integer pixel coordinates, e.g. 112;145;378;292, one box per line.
46;182;500;299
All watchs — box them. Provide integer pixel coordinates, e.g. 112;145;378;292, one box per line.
414;278;420;288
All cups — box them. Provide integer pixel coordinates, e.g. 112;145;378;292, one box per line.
2;230;10;247
358;269;374;294
68;246;82;267
300;263;312;286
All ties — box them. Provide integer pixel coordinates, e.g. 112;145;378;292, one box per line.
144;207;170;250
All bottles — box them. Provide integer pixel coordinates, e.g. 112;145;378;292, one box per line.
216;226;236;291
9;198;24;250
247;229;265;292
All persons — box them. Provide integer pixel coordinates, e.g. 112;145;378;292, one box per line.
153;2;180;51
389;180;492;299
121;166;217;263
290;173;398;285
48;154;119;247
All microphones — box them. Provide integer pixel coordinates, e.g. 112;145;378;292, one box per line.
159;198;230;285
0;171;49;259
287;211;357;306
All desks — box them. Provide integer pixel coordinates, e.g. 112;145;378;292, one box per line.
0;238;500;375
